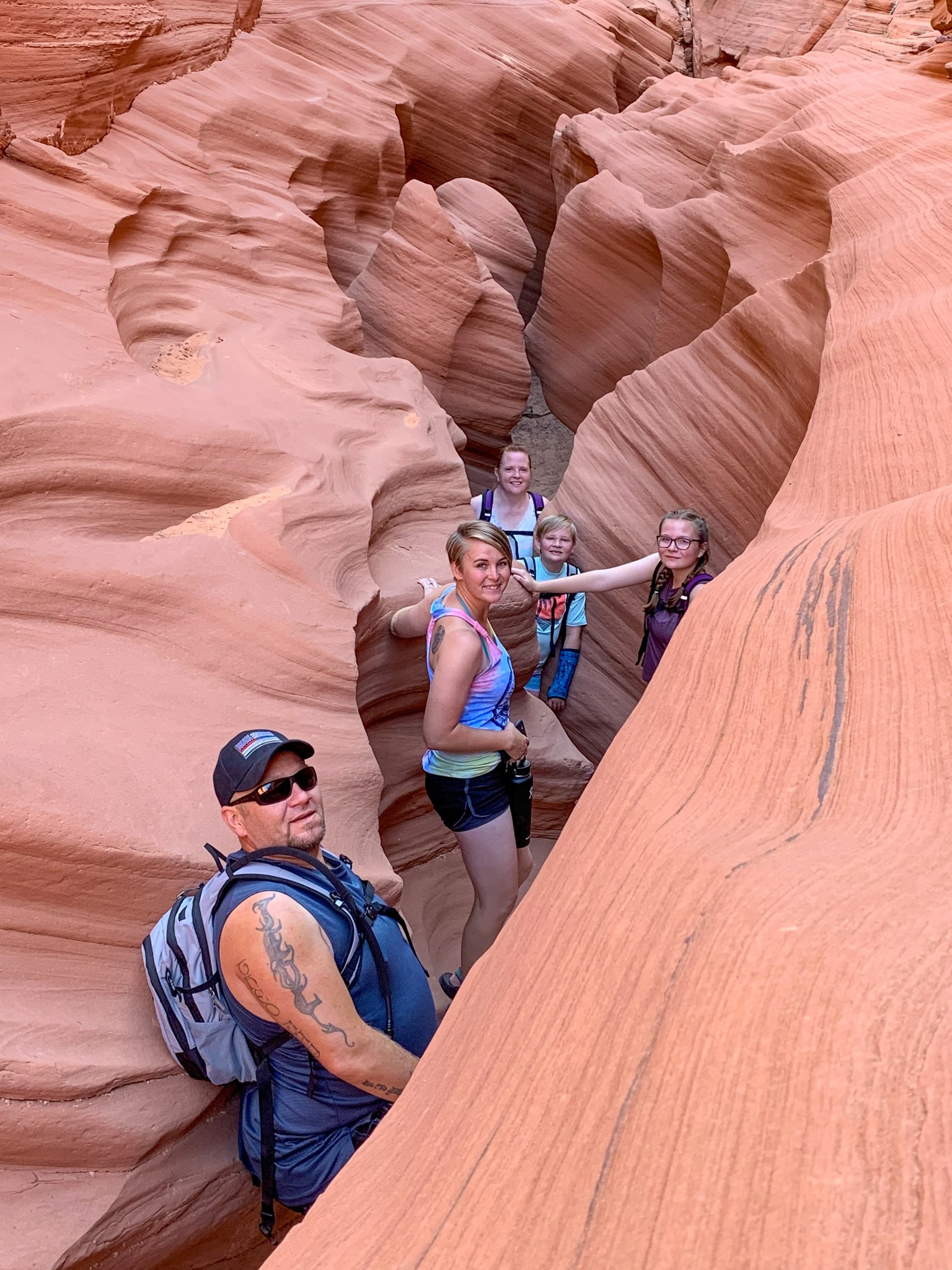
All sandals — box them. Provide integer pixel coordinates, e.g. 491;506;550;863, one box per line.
439;967;463;1001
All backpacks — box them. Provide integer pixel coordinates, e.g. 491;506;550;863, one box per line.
141;843;375;1087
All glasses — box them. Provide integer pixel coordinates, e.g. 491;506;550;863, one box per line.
228;766;317;807
656;535;702;550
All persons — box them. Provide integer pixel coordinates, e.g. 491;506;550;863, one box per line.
517;514;586;711
511;509;716;683
390;520;533;1000
471;443;548;559
213;729;440;1215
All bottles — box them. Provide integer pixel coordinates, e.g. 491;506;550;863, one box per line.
506;754;531;849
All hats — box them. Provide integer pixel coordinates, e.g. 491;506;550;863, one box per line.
213;729;315;807
